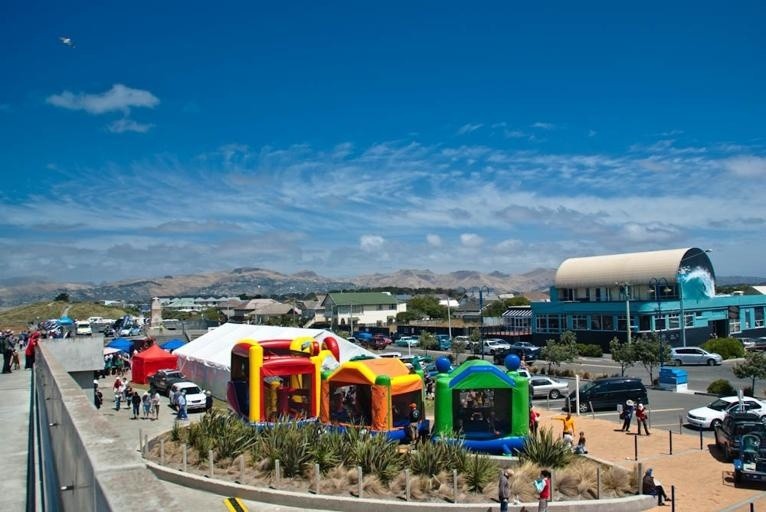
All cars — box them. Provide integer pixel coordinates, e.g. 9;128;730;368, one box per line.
686;394;766;487
167;381;207;413
350;328;571;400
41;316;150;337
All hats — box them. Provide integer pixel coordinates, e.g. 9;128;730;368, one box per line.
409;403;417;408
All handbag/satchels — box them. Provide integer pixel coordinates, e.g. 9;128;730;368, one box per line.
620;412;625;419
575;446;584;454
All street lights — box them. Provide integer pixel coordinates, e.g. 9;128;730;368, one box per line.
612;279;632;342
468;284;492;360
646;275;674;368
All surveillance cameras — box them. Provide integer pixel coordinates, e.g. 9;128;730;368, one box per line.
499;294;515;302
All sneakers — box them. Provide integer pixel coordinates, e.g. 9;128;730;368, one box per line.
621;429;650;435
658;497;671;505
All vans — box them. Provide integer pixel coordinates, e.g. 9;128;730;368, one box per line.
669;336;766;367
564;376;648;414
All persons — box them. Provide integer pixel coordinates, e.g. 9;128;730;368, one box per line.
642;468;672;506
499;468;515;512
521;355;529;369
0;320;76;374
622;399;634;432
424;374;434;400
93;344;214;421
533;469;551;512
636;403;650;435
456;388;500;433
529;399;588;456
408;403;421;444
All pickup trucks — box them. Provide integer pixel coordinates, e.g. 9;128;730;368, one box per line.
147;366;191;397
